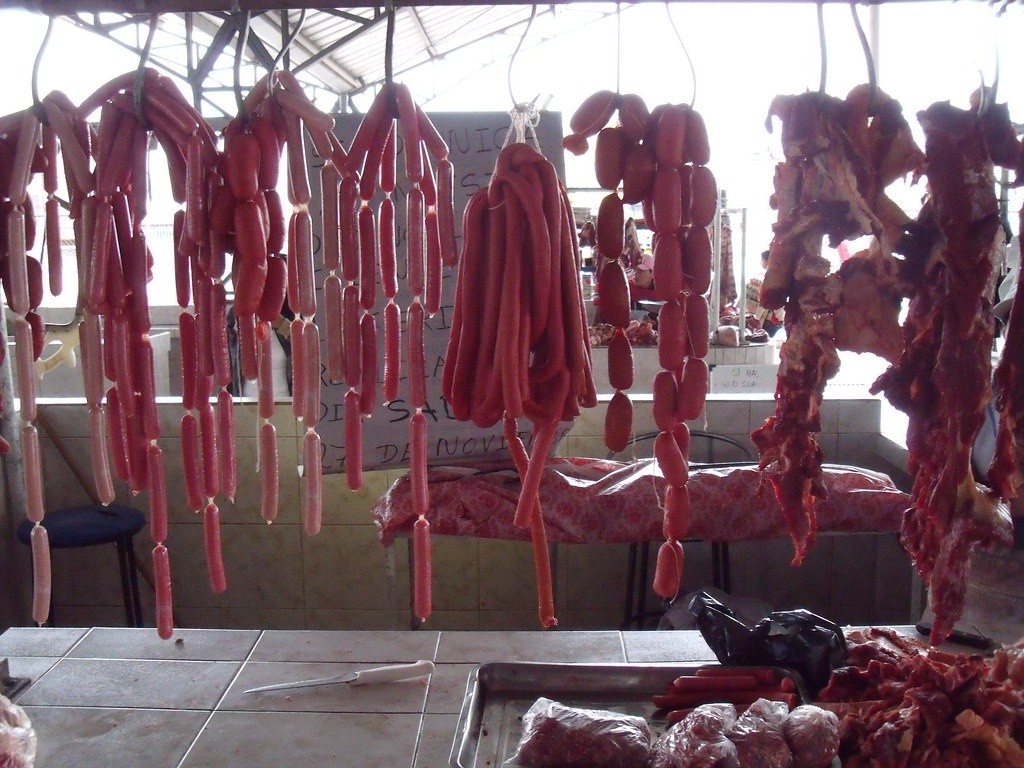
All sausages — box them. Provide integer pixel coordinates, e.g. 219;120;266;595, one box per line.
652;665;798;723
0;68;718;628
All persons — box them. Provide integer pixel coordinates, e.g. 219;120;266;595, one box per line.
754;249;786;337
210;250;317;399
632;255;659;331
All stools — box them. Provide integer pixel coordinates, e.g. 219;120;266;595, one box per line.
17;502;146;627
606;429;750;629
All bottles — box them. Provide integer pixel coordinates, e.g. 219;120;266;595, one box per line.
580;266;595;301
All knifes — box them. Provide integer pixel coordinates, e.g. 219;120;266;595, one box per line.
917;621;1003;656
241;659;436;694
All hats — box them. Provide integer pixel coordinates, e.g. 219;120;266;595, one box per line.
636;254;653;272
